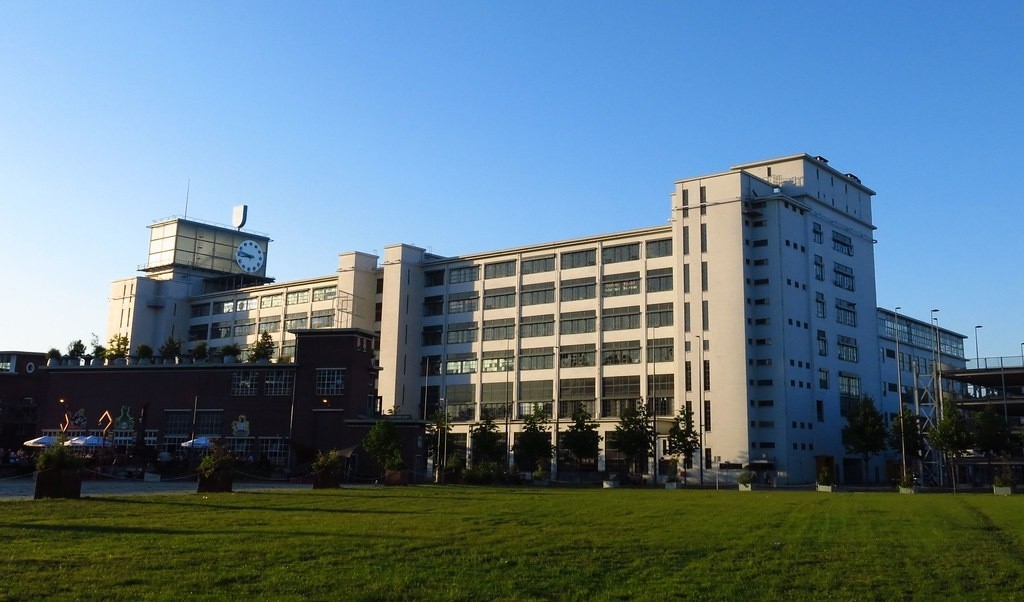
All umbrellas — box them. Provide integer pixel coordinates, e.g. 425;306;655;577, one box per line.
23;436;113;456
181;436;214;448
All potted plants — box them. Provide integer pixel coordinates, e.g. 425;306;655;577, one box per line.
195;444;234;494
33;433;85;500
737;465;753;492
310;450;341;489
992;475;1011;495
602;472;619;489
897;467;914;494
383;454;410;485
663;473;682;490
530;464;551;487
816;464;834;492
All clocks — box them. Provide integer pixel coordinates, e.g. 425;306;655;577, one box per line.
235;240;263;273
